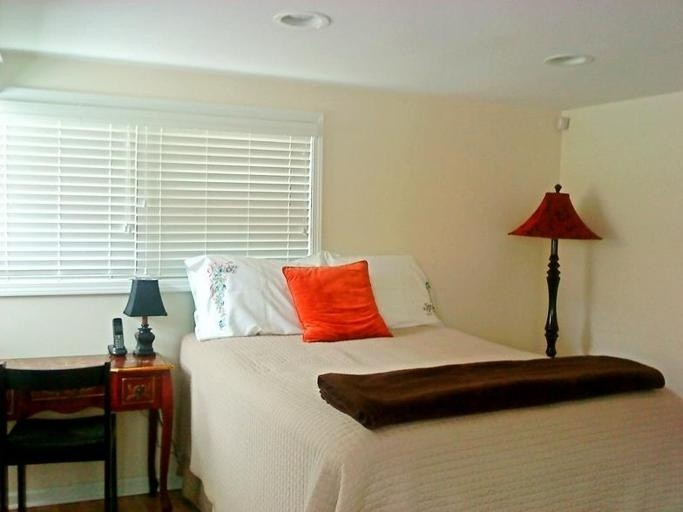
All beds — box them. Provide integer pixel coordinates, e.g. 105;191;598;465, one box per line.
175;327;682;512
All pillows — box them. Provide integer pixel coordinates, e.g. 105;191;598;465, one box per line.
282;260;394;342
325;252;439;329
185;255;329;342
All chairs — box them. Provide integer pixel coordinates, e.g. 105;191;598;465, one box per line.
0;362;116;512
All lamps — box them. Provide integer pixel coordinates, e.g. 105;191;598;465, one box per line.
124;280;167;356
507;185;602;357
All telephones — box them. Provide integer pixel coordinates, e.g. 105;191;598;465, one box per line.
108;318;127;356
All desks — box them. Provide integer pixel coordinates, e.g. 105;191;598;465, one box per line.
0;353;173;512
